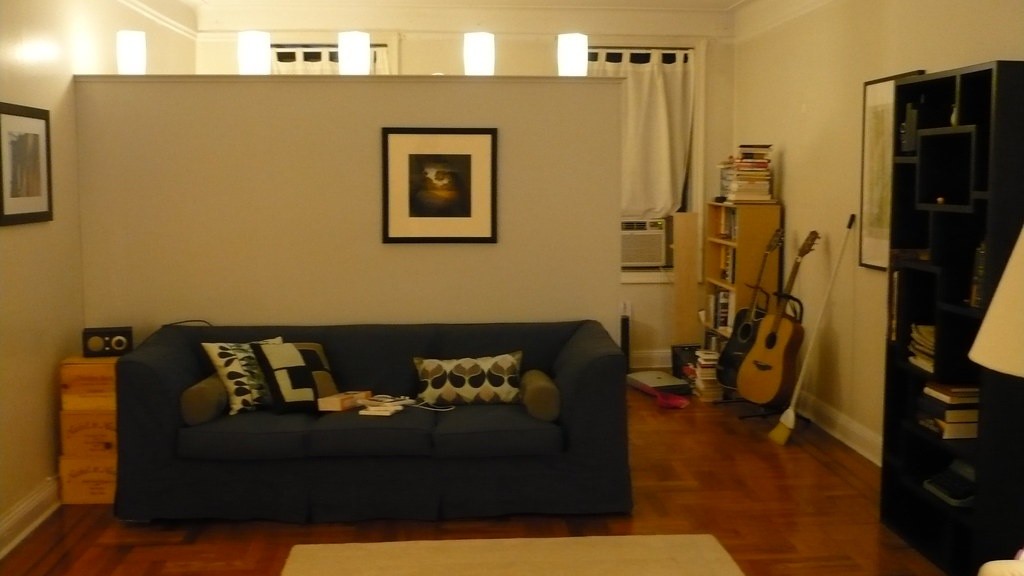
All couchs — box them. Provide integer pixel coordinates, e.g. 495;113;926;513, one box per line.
112;318;633;524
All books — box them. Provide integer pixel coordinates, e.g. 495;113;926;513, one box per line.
720;207;737;241
908;323;936;373
693;331;729;403
720;245;736;285
722;144;779;206
919;381;979;439
359;395;415;417
709;289;734;332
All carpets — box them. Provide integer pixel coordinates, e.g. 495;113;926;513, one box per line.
280;535;745;576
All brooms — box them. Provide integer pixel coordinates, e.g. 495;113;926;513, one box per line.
767;213;856;447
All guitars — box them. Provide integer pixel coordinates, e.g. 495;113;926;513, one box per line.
715;227;785;390
736;229;821;408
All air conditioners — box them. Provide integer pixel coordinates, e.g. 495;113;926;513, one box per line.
621;219;667;269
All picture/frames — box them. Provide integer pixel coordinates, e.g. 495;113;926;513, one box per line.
381;125;499;246
0;101;54;227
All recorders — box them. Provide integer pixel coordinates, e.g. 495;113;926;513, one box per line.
82;326;134;358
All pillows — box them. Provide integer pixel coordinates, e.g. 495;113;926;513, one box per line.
201;344;269;417
251;341;342;412
415;351;524;407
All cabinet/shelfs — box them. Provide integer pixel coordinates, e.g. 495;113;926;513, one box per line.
60;356;120;499
878;59;1023;576
699;198;783;363
